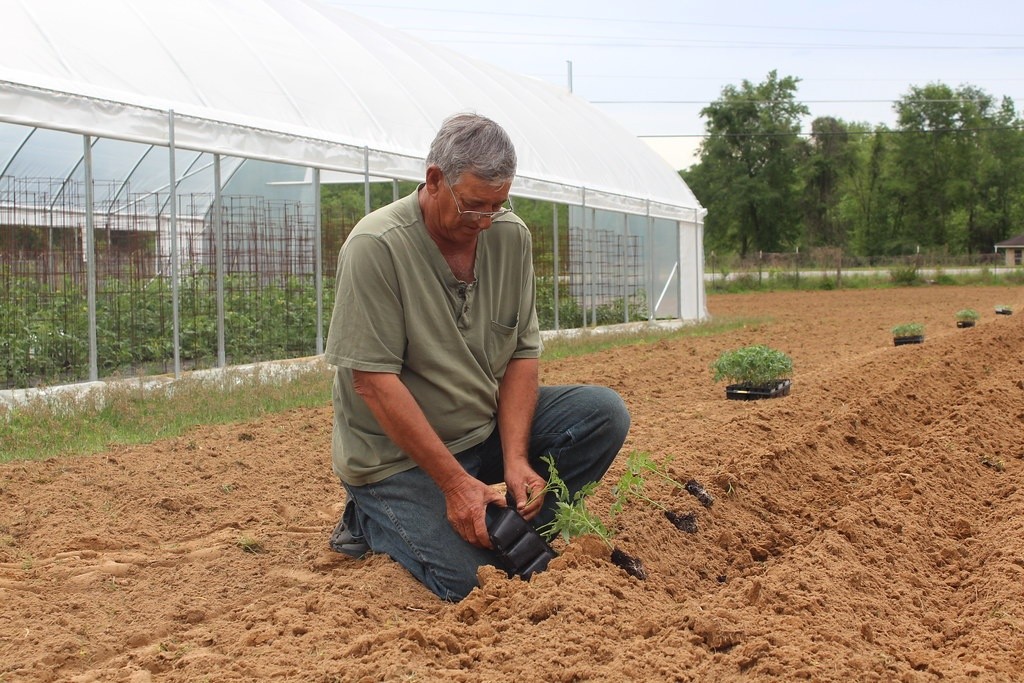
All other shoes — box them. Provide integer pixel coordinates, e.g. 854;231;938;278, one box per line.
329;492;368;557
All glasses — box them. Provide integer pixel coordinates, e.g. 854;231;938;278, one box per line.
442;170;514;223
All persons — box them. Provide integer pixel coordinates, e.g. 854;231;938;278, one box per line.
323;113;630;603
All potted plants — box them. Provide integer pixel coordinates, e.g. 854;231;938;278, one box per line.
707;344;793;401
892;322;925;346
993;305;1013;315
488;451;573;583
954;309;980;328
